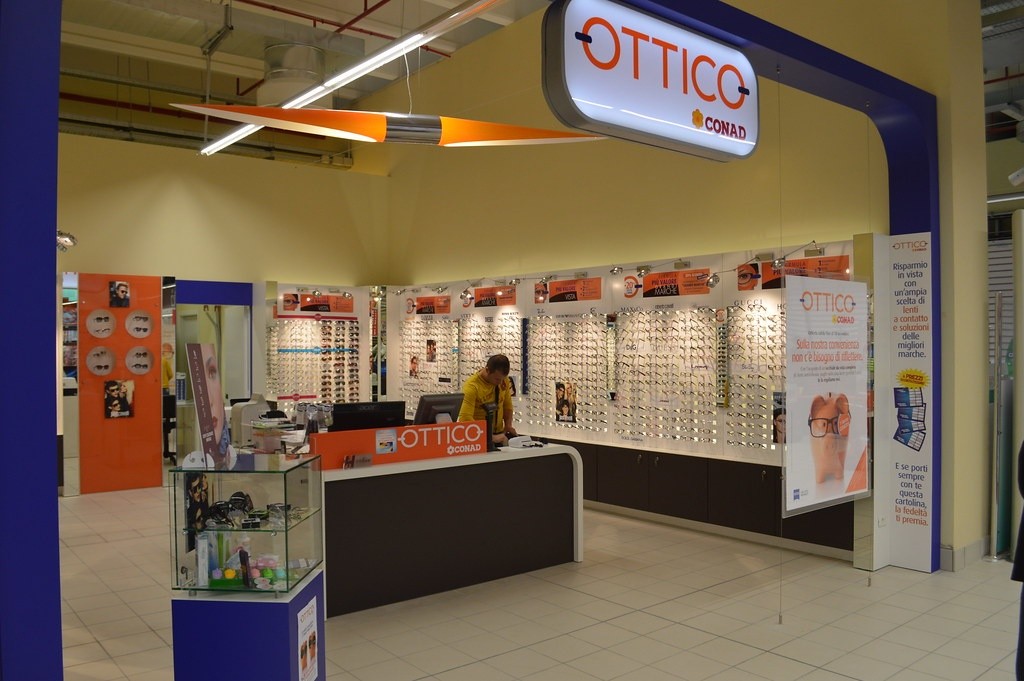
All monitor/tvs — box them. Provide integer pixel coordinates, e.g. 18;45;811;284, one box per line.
413;393;465;425
332;401;406;431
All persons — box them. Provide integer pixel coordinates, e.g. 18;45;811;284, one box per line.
161;343;175;395
457;354;518;446
1010;440;1024;681
556;382;577;422
284;294;300;311
737;263;761;290
427;342;436;362
185;472;208;552
110;283;130;307
773;407;787;444
535;283;547;303
104;380;134;417
199;343;230;444
410;356;418;376
508;376;517;396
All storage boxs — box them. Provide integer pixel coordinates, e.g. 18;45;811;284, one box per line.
184;342;231;468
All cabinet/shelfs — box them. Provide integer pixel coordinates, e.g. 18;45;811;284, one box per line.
166;451;326;681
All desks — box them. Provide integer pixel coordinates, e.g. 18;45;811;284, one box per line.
321;441;585;621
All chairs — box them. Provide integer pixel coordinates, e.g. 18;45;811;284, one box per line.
163;394;177;467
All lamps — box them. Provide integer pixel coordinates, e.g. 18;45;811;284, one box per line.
610;264;651;275
329;287;352;299
432;286;448;296
297;287;322;298
200;0;496;157
460;277;505;300
395;287;420;296
770;239;823;271
509;276;556;286
168;0;612;147
637;257;691;279
706;253;774;287
539;271;587;284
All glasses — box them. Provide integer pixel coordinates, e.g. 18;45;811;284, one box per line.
96;364;109;369
111;401;120;407
807;411;852;437
119;290;128;294
133;351;147;357
191;482;203;493
132;326;148;332
195;514;204;523
109;388;119;392
131;363;148;368
132;316;149;322
263;305;786;459
94;327;111;333
93;316;109;322
207;490;303;529
